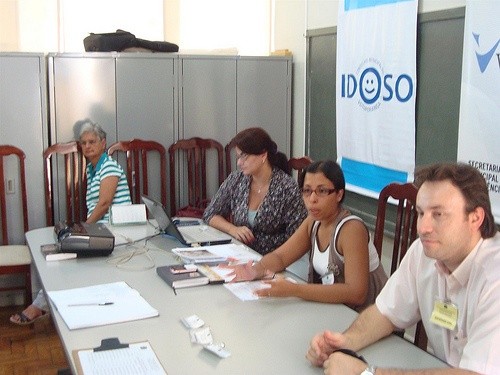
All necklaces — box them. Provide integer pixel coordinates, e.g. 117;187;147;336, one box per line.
254;182;266;194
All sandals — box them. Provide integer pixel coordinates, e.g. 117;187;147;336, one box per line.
9;307;49;324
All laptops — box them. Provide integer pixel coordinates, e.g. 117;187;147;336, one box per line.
141;194;232;246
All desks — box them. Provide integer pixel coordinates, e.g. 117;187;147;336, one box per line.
25;217;451;375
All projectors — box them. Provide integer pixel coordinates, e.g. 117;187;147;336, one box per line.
53;222;114;256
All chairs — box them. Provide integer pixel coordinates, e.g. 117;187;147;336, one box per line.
0;137;428;351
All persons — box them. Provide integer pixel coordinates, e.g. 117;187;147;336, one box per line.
10;122;132;325
305;163;500;375
224;161;388;314
202;128;308;256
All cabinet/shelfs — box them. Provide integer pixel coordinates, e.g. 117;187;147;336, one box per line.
178;55;293;208
0;51;51;306
46;51;180;227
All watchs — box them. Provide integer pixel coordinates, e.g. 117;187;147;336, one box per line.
360;365;376;375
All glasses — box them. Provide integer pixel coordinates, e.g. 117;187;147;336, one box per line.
235;152;248;162
302;188;336;197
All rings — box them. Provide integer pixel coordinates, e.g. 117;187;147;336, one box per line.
268;292;270;296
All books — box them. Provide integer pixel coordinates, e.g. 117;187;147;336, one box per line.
156;264;209;289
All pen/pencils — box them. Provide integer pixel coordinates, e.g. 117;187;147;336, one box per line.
69;301;114;307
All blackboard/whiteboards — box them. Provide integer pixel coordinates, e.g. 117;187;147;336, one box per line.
306;4;467;185
